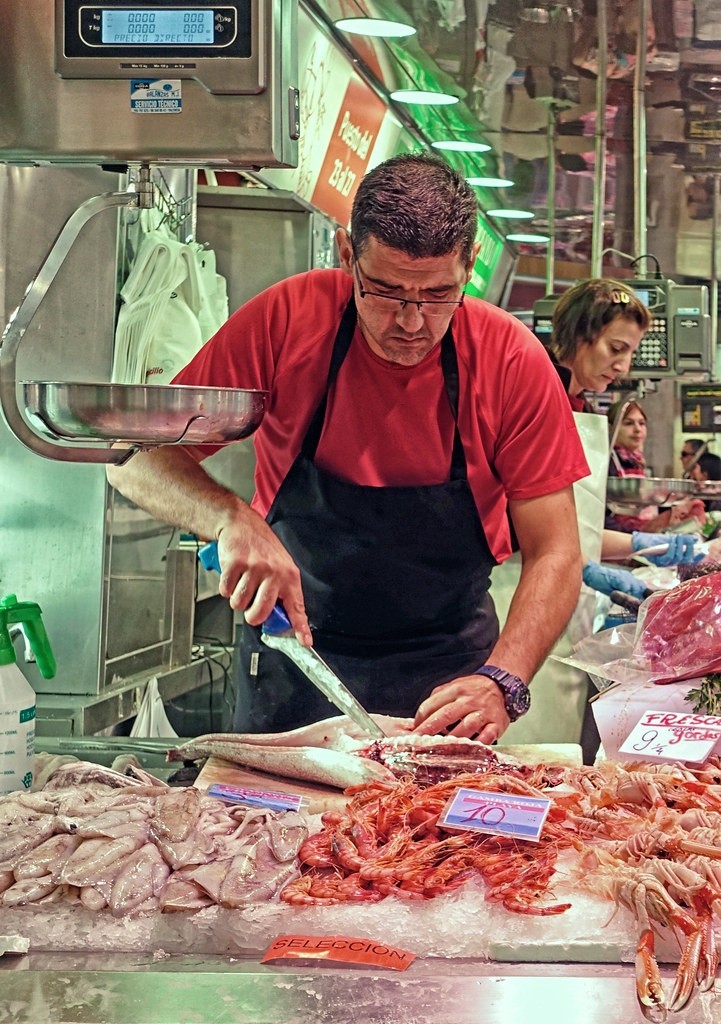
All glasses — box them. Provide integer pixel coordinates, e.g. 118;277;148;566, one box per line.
681;450;696;457
350;234;473;314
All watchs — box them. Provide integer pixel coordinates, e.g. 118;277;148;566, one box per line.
474;666;530;723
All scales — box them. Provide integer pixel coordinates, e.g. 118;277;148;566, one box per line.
1;2;311;467
533;253;721;510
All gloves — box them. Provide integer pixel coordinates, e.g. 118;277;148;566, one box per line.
632;530;706;568
582;559;646;598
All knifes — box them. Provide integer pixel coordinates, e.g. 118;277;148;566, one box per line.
198;541;385;739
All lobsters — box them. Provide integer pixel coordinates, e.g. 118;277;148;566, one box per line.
565;754;721;1013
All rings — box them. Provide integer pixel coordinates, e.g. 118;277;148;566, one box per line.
475;712;484;722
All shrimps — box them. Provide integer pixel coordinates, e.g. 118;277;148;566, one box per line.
280;764;584;916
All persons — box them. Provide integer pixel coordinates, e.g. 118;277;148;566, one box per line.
602;402;705;536
489;279;706;746
106;153;592;747
680;439;721;511
